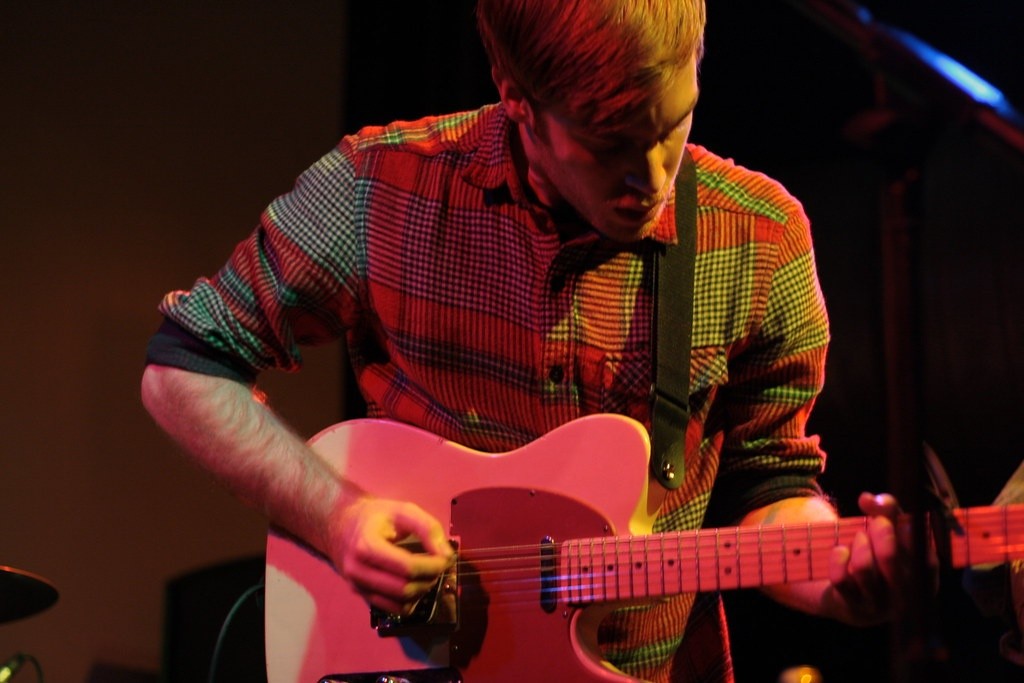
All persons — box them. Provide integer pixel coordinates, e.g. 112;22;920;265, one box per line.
141;1;931;683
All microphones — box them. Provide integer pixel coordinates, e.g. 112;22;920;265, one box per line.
1;651;26;683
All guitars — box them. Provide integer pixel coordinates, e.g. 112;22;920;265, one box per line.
260;411;1024;683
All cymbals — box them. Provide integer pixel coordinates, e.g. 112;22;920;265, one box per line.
0;563;60;625
